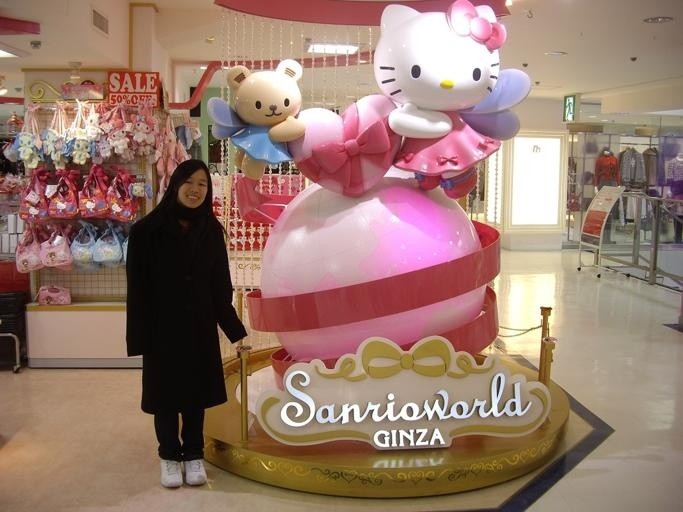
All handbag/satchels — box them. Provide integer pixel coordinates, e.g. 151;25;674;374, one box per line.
38;286;71;305
16;225;127;273
19;168;137;222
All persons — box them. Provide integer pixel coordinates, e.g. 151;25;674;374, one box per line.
125;158;248;489
564;98;574;121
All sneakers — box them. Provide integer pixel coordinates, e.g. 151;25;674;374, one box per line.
186;459;208;486
159;459;184;488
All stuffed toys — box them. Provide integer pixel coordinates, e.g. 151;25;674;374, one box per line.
17;112;159;171
